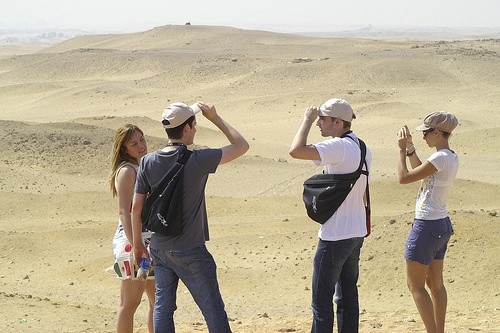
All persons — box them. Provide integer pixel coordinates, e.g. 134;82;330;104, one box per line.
132;102;250;333
397;111;459;333
289;98;371;333
109;124;157;333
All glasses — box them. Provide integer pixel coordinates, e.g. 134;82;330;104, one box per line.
423;129;434;136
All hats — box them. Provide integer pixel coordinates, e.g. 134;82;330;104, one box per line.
161;102;201;129
317;99;354;122
415;111;458;133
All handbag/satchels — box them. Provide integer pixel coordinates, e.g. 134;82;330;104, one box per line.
141;150;193;236
303;173;359;224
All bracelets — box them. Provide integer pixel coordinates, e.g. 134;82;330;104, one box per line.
406;144;415;156
